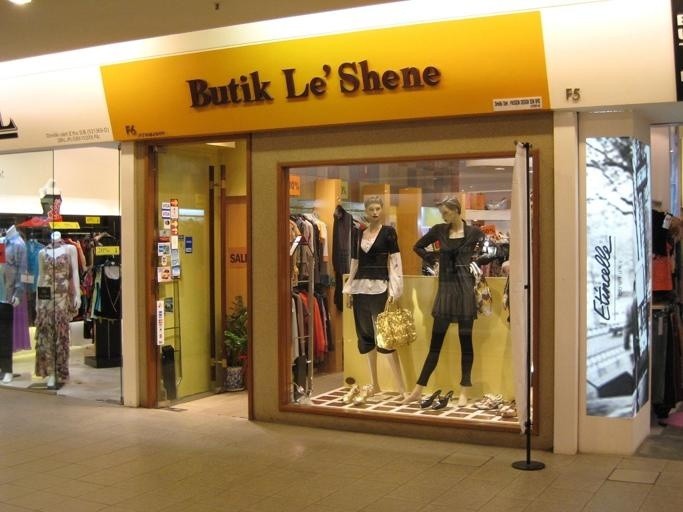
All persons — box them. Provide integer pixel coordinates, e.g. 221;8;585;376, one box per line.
401;193;503;407
79;231;97;340
339;197;410;403
32;231;83;388
0;223;27;385
39;177;63;220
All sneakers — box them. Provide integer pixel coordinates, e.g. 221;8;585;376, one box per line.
475;394;518;418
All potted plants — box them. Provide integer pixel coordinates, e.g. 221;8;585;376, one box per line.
222;294;250;390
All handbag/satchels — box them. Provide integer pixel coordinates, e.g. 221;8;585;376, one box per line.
376;309;417;350
474;275;492;316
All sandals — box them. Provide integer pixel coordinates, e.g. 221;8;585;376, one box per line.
343;384;374;406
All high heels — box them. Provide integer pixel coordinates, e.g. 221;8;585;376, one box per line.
433;391;453;410
422;390;442;408
